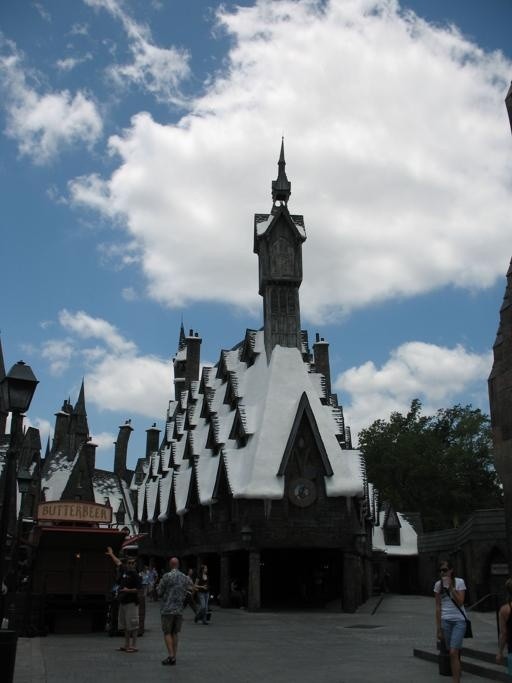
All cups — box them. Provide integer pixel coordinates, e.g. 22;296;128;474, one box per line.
438;568;452;573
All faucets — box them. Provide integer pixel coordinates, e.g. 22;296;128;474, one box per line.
162;655;177;667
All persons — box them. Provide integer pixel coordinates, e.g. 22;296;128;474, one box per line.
495;577;512;683
104;545;140;653
433;560;469;683
138;563;209;625
155;557;193;665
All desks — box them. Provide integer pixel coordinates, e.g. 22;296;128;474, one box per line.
1;359;39;631
15;465;32;578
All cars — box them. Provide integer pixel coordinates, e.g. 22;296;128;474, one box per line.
442;576;450;588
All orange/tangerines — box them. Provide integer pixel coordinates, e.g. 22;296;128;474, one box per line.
188;589;214;624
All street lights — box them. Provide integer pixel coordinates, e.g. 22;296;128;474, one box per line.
463;619;474;640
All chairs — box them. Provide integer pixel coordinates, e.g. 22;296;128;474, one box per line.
194;616;209;626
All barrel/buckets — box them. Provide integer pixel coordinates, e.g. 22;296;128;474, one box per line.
114;645;139;654
